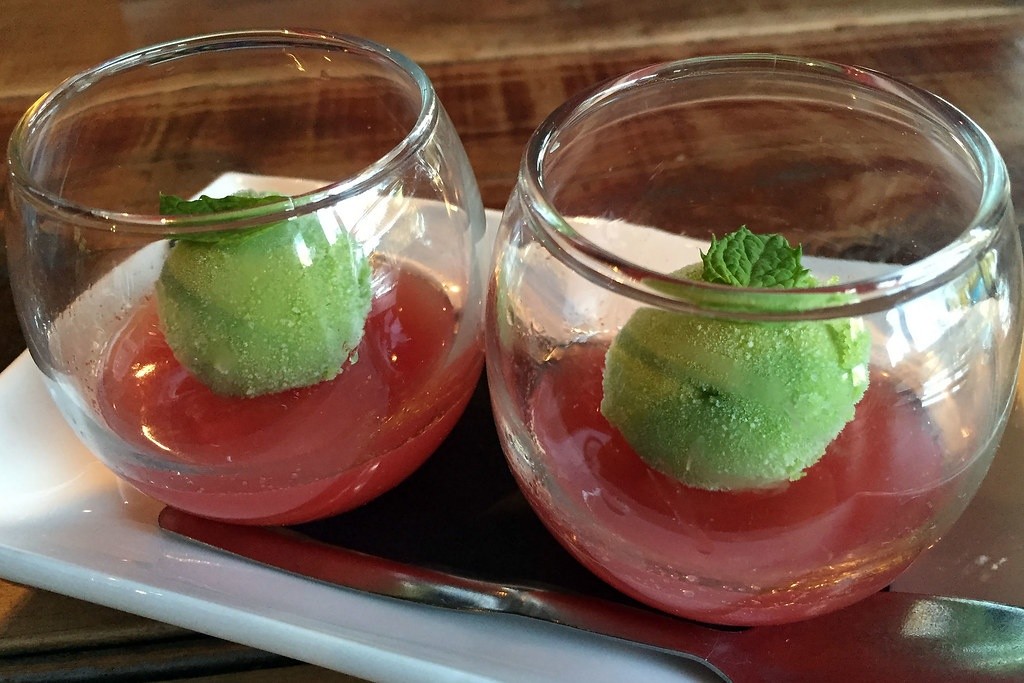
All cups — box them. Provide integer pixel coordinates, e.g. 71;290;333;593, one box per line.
0;27;488;527
484;54;1024;630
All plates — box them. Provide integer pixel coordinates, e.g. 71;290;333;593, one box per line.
0;174;1024;683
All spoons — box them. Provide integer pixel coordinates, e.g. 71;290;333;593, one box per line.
155;504;1024;683
